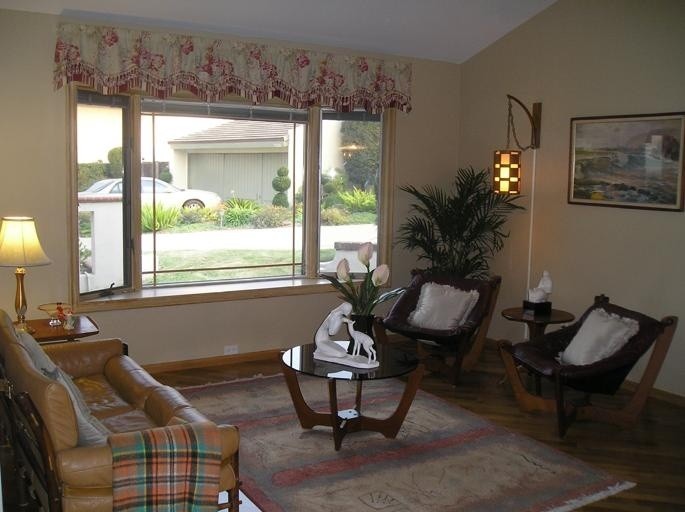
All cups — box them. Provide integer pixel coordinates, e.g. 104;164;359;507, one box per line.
64;313;77;331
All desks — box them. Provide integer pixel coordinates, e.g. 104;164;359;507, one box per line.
501;305;575;398
2;314;98;347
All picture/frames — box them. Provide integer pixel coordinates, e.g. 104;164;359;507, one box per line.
568;109;684;213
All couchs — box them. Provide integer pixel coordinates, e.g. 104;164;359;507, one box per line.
1;309;240;511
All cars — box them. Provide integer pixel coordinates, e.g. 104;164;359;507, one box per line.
76;175;223;215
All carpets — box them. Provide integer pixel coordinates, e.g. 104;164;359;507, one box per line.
171;369;638;511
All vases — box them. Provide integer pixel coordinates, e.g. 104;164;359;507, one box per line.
346;312;377;353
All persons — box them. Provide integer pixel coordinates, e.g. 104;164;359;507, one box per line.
311;300;353;359
526;269;552;303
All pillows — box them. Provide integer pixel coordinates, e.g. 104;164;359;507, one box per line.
18;330;58;380
47;367;114;447
407;280;482;331
557;308;640;368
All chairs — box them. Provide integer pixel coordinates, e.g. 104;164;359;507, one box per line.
379;268;502;380
514;292;678;437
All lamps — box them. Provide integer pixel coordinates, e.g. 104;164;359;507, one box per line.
491;94;543;345
1;217;53;333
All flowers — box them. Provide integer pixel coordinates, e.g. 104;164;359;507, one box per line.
318;242;409;317
